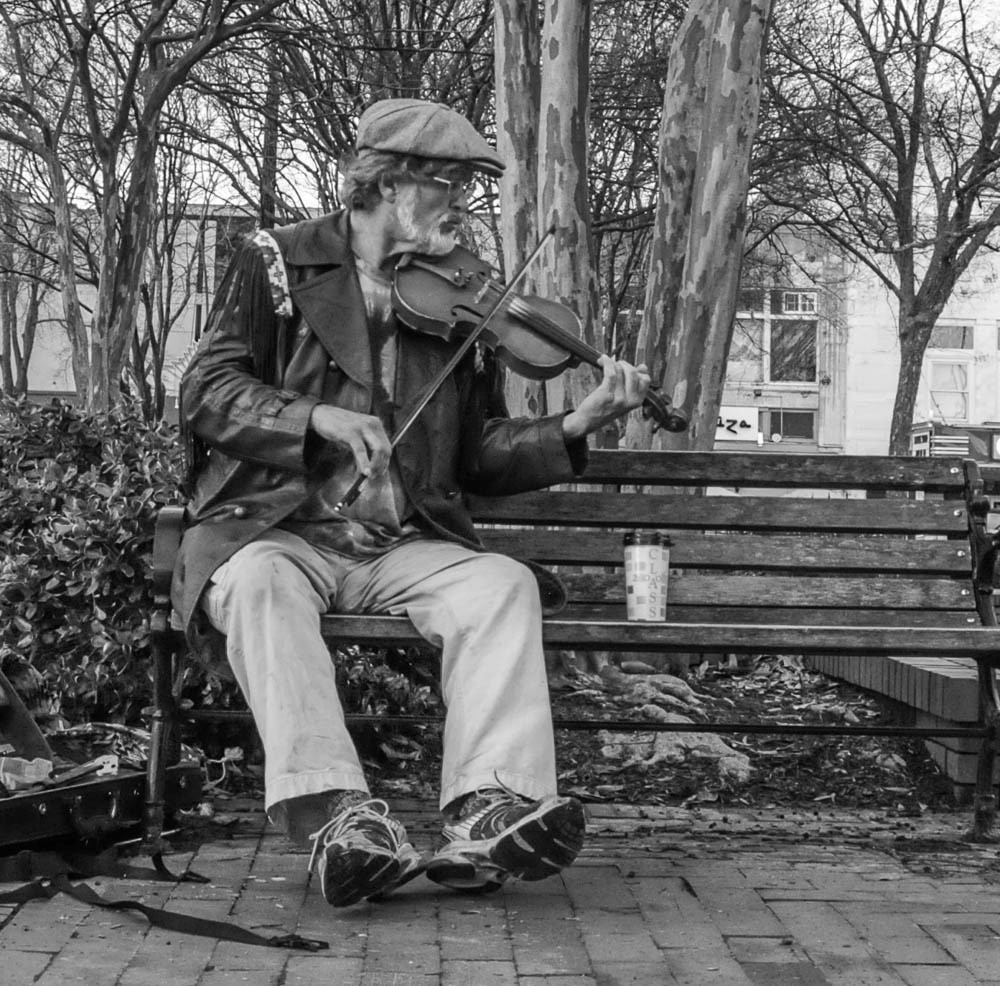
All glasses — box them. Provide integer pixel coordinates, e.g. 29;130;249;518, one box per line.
414;173;475;200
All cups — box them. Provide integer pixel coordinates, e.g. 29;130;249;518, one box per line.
623;532;675;622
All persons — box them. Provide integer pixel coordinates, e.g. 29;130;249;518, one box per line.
175;100;652;910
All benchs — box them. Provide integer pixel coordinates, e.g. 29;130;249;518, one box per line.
137;447;1000;854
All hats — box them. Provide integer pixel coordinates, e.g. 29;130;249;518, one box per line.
355;97;507;178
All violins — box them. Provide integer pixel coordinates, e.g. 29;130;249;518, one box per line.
392;245;691;436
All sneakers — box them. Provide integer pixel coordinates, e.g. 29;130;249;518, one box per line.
309;798;429;907
427;769;586;895
